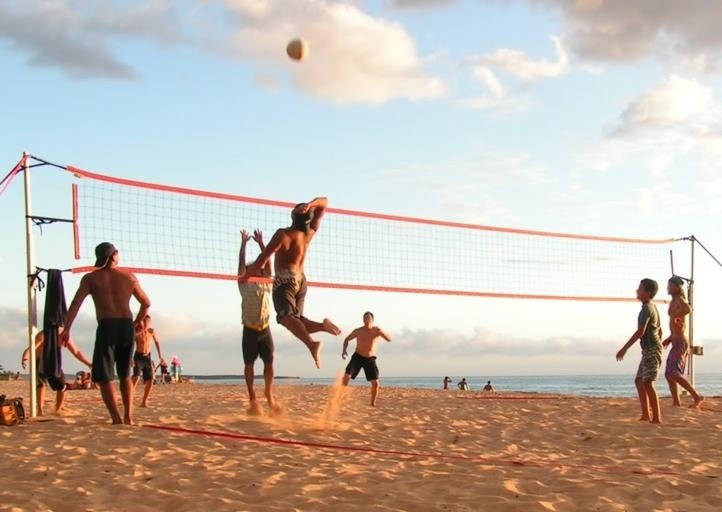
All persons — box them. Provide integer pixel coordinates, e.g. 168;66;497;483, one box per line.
8;242;193;425
238;227;275;411
483;381;494;392
443;376;452;389
342;311;391;406
662;275;705;409
238;197;342;369
457;378;468;390
616;278;664;425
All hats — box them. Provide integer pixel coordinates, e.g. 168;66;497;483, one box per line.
295;203;315;232
92;242;116;270
669;276;687;299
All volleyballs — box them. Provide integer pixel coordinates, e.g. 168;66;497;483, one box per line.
286;37;308;61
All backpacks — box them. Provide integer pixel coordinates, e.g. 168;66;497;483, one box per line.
0;395;25;426
